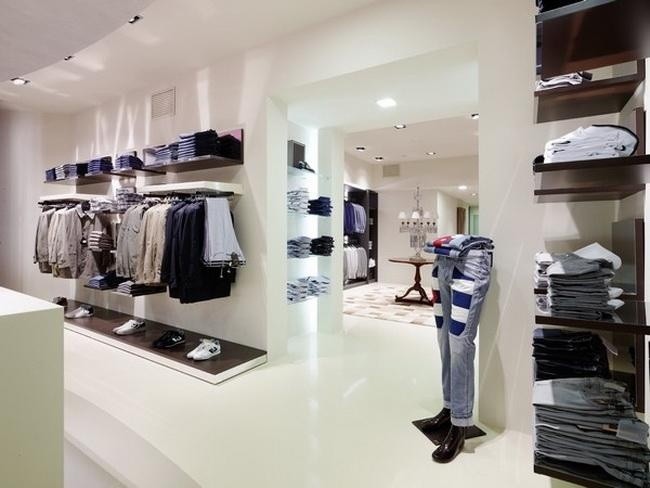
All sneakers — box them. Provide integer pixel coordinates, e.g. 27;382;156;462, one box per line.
186;338;222;362
64;304;95;319
152;328;186;350
112;318;147;336
51;295;68;309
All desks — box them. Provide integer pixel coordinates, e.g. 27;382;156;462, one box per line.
389;257;437;307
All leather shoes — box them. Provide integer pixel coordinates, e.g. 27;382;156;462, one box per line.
421;407;451;433
432;426;465;464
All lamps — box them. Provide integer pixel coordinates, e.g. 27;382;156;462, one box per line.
398;183;438;247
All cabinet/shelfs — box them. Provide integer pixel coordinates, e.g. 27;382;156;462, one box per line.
38;128;244;203
287;165;334;307
348;189;378;284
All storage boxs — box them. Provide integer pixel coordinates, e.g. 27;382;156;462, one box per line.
432;277;441;303
